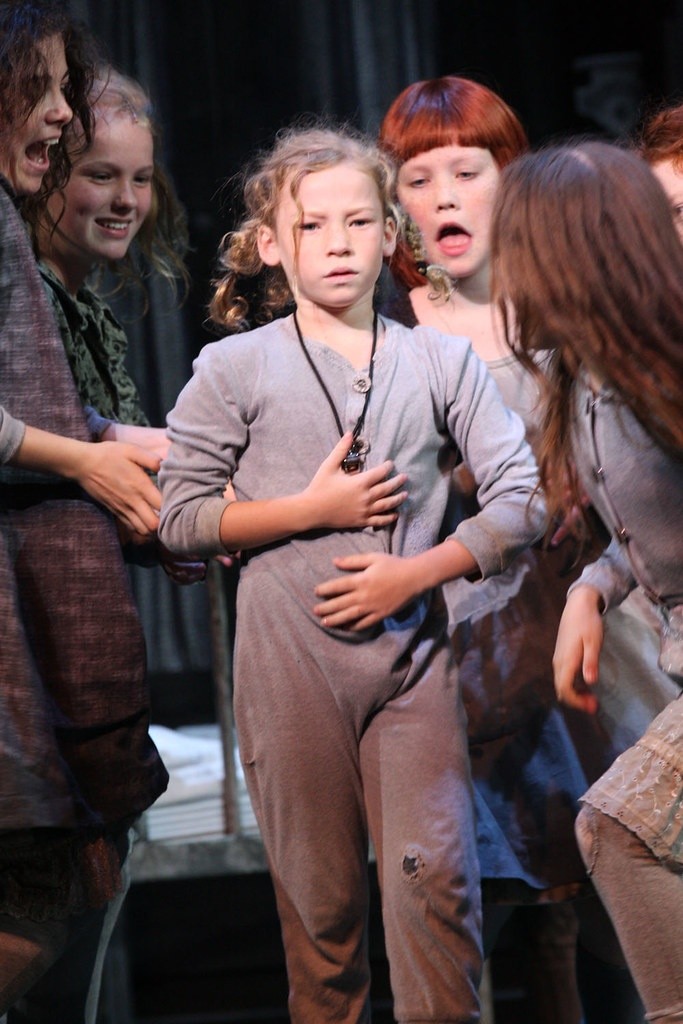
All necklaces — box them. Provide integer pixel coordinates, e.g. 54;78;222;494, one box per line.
293;314;378;475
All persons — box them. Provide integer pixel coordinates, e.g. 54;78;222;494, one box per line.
489;105;683;1024
0;0;243;1023
31;60;243;1024
379;77;588;1023
158;125;549;1023
